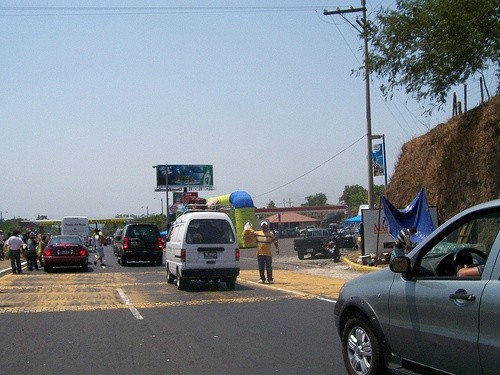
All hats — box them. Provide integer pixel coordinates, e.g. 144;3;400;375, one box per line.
261;221;268;226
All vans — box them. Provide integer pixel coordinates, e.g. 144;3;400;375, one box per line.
165;211;241;290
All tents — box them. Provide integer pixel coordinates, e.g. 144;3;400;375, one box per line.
343;215;362;228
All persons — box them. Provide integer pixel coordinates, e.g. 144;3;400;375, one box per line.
456;264;485;277
254;221;279;284
0;229;51;274
390;226;417;259
84;228;106;266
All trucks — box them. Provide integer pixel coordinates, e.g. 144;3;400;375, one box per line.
36;214;47;220
62;216;89;245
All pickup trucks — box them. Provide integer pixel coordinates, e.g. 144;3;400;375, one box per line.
294;228;335;260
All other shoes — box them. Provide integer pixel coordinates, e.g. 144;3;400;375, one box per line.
94;261;96;266
270;280;273;284
100;264;106;266
258;280;264;283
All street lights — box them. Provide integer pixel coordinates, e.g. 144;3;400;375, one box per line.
1;211;8;221
141;205;148;218
153;198;163;214
372;134;387;185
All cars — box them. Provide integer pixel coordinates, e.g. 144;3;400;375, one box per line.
333;198;500;375
275;226;329;237
41;235;89;272
112;224;163;267
